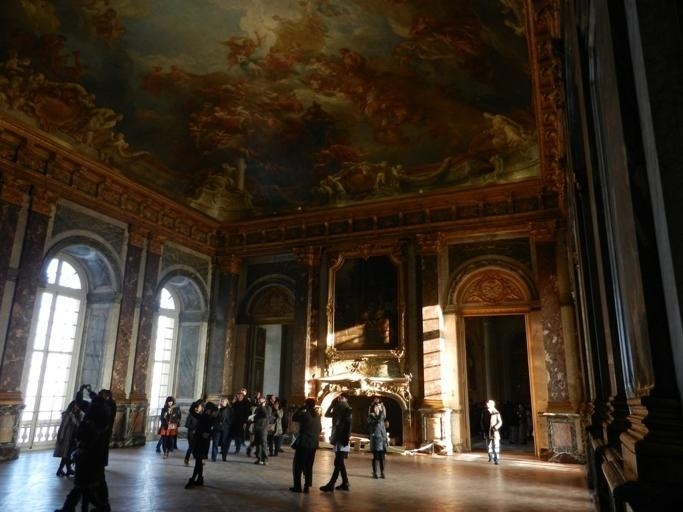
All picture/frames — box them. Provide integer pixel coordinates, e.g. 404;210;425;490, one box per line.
321;240;409;361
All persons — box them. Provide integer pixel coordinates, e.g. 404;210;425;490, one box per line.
481;400;503;465
368;394;387;422
320;392;354;492
53;384;118;512
184;387;287;491
289;398;322;494
156;396;182;459
367;405;389;479
516;402;528;444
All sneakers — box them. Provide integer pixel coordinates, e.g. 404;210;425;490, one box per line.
197;476;203;485
54;507;76;512
184;461;191;466
67;470;74;476
156;448;181;457
335;484;350;490
320;483;334;491
185;478;196;488
373;473;378;479
379;473;385;478
289;486;302;492
304;485;309;492
56;470;65;476
202;449;285;465
